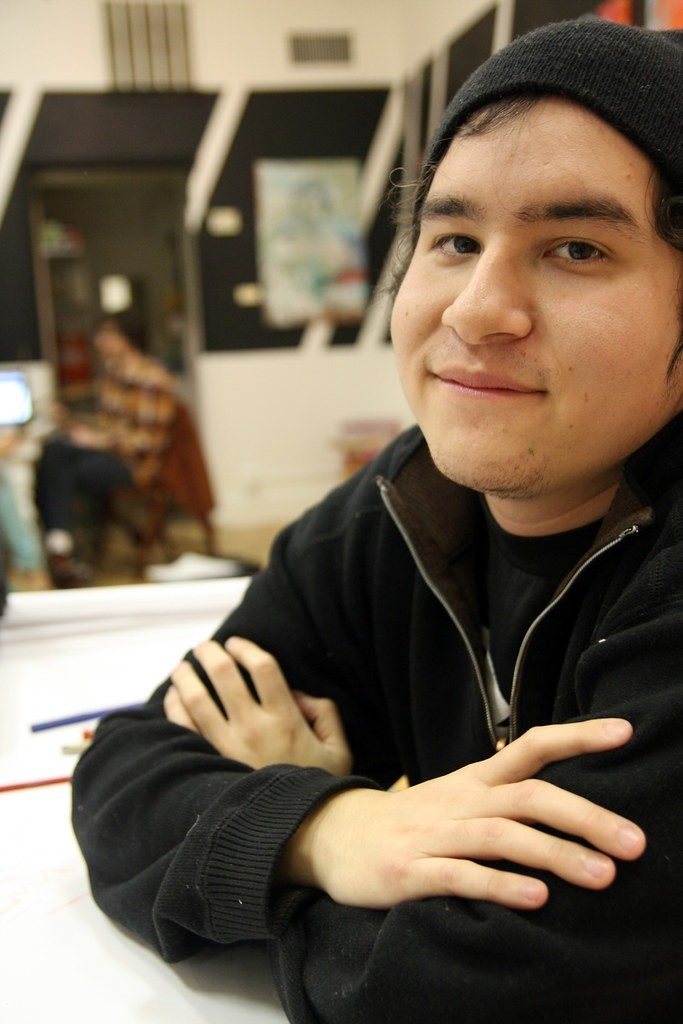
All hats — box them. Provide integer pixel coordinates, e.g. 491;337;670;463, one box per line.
414;19;682;271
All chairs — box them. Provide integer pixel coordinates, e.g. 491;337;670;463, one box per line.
99;393;217;579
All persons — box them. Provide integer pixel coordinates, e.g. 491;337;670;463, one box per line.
72;19;683;1024
35;315;182;582
